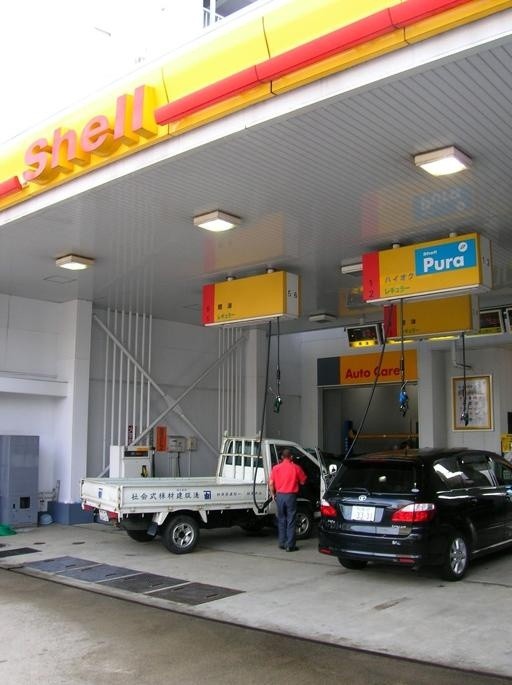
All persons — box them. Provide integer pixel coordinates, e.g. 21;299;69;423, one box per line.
267;448;307;553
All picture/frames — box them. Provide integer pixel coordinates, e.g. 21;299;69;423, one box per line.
451;373;494;432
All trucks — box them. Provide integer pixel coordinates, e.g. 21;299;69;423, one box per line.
78;431;333;551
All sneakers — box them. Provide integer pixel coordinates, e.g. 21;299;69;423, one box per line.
279;544;300;552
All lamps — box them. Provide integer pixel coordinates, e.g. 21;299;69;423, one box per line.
54;254;95;272
308;314;337;324
193;211;241;232
414;145;474;177
341;262;363;277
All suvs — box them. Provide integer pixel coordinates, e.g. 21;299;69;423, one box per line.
309;444;510;581
